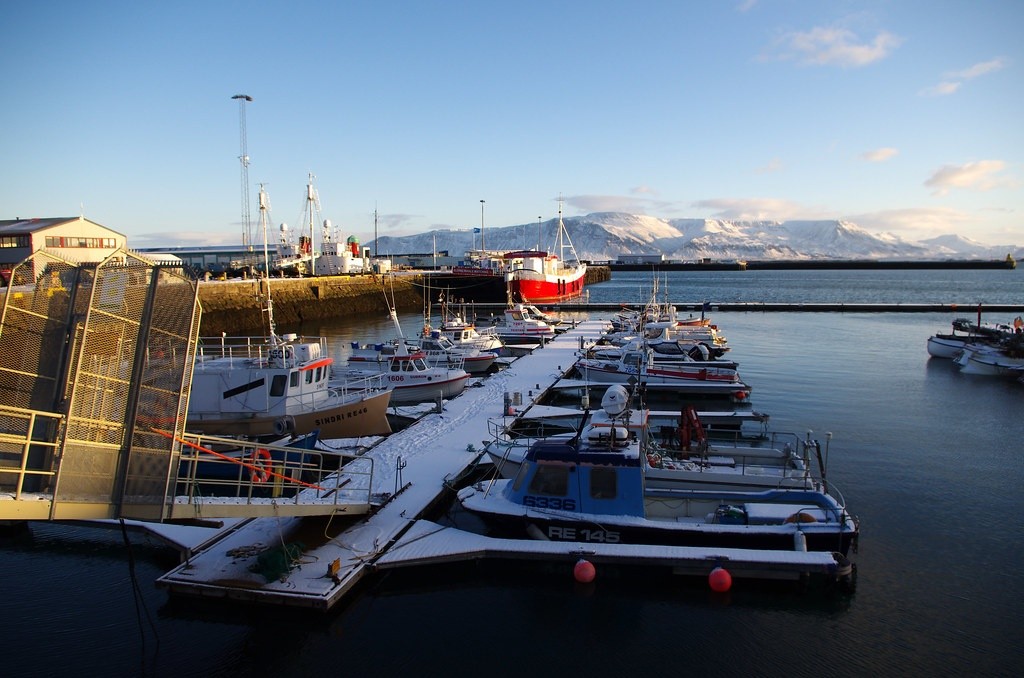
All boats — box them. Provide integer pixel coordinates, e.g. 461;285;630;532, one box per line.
454;265;859;552
347;325;499;375
926;319;1024;374
328;305;471;402
499;192;587;304
492;303;562;326
84;299;398;438
436;317;505;358
129;428;320;496
474;304;555;336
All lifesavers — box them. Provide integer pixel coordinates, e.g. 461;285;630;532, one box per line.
248;449;272;483
785;514;817;522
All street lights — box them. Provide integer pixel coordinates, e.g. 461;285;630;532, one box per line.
480;200;486;256
538;216;541;250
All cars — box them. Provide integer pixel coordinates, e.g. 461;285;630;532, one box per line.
0;265;25;287
